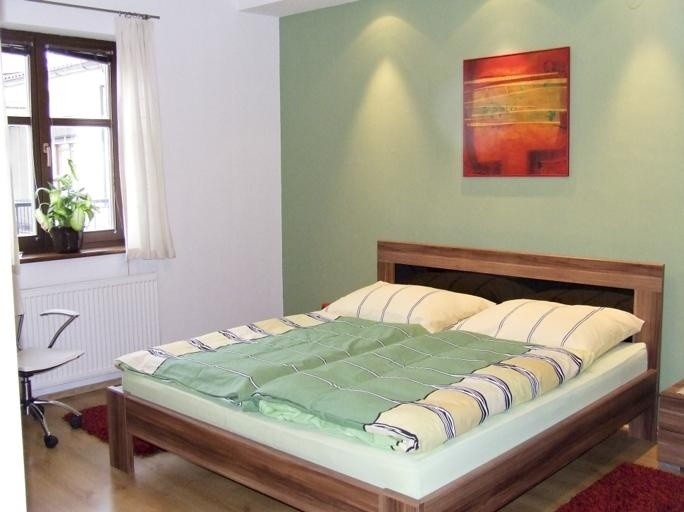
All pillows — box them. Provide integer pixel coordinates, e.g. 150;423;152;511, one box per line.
451;297;645;372
318;280;496;334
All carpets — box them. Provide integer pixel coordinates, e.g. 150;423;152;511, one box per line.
62;404;166;457
554;461;684;512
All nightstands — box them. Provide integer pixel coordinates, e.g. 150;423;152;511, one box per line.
657;379;684;470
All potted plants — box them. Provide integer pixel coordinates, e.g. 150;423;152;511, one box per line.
35;158;99;253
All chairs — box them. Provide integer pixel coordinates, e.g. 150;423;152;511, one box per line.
15;309;83;448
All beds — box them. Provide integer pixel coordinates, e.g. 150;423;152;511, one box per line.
105;241;665;512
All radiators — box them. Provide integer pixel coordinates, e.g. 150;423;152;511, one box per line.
20;273;161;390
